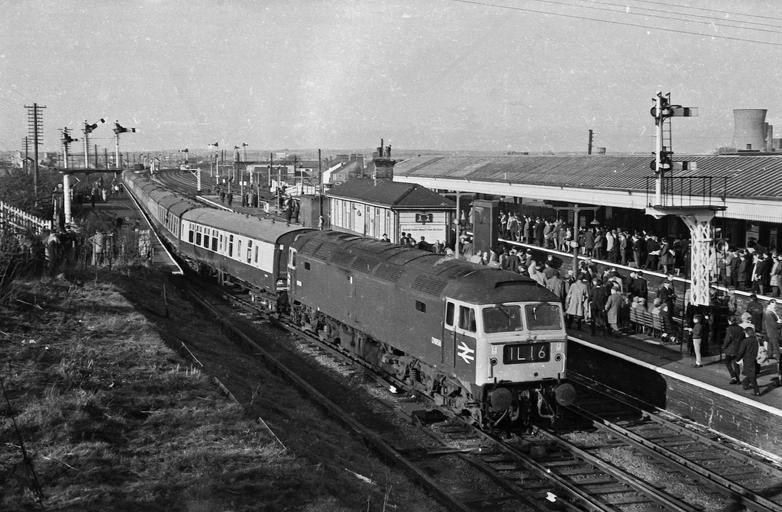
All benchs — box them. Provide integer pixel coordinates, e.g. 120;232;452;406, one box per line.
629;307;675;338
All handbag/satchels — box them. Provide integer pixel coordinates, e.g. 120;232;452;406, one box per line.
756;363;761;375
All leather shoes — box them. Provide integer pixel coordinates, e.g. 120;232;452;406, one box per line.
743;385;761;396
729;378;741;385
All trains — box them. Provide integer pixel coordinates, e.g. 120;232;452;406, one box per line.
116;168;576;438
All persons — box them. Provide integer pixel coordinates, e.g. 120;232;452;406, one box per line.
721;316;745;385
214;182;300;224
684;314;702;370
382;200;781;338
44;215;141;271
88;178;123;208
735;326;761;398
763;299;782;361
